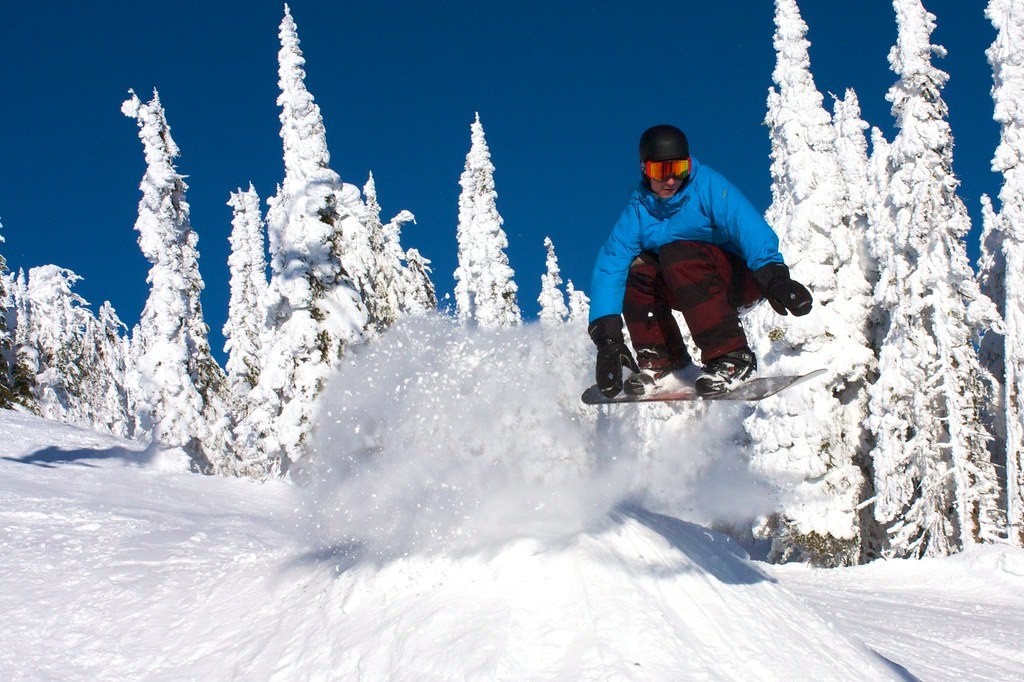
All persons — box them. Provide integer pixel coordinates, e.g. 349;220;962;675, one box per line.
587;123;813;398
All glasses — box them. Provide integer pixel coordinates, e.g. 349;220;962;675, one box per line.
642;157;692;182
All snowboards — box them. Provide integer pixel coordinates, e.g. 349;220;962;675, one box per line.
583;368;824;403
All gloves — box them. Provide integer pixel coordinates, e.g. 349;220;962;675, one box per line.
752;262;813;317
588;315;641;399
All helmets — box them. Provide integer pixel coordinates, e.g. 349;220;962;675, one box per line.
639;125;690;161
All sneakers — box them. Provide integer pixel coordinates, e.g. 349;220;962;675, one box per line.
624;349;693;395
694;345;757;400
